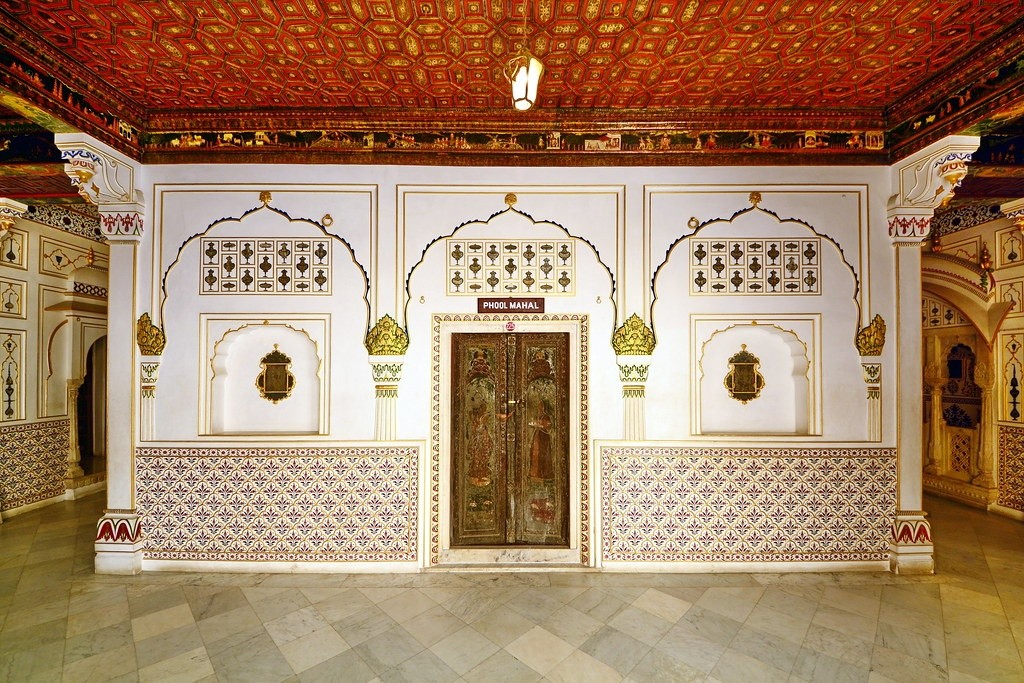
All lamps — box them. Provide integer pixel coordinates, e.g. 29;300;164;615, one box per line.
503;0;546;113
